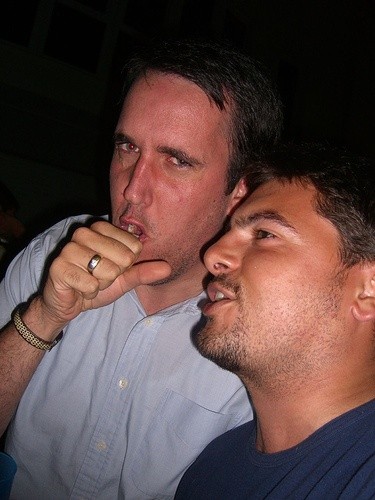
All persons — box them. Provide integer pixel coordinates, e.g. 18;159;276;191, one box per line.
175;146;375;500
0;40;283;500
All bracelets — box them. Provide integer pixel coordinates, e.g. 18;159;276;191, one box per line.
11;302;64;352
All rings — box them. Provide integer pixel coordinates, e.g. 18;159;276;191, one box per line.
87;253;104;274
